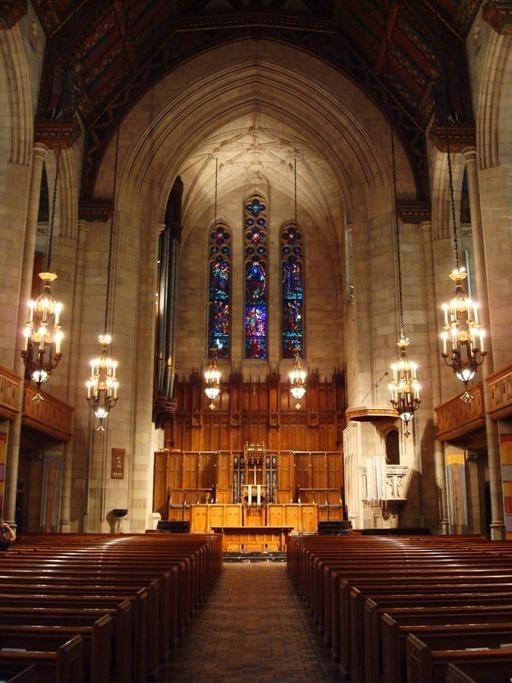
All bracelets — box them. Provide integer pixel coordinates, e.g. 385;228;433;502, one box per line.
7;526;11;530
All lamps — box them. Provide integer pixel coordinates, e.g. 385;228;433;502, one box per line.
387;122;488;438
20;113;118;441
197;156;311;415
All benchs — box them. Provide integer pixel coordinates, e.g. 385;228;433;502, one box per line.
284;528;510;679
1;530;227;681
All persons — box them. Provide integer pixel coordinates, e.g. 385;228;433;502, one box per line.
1;522;18;550
